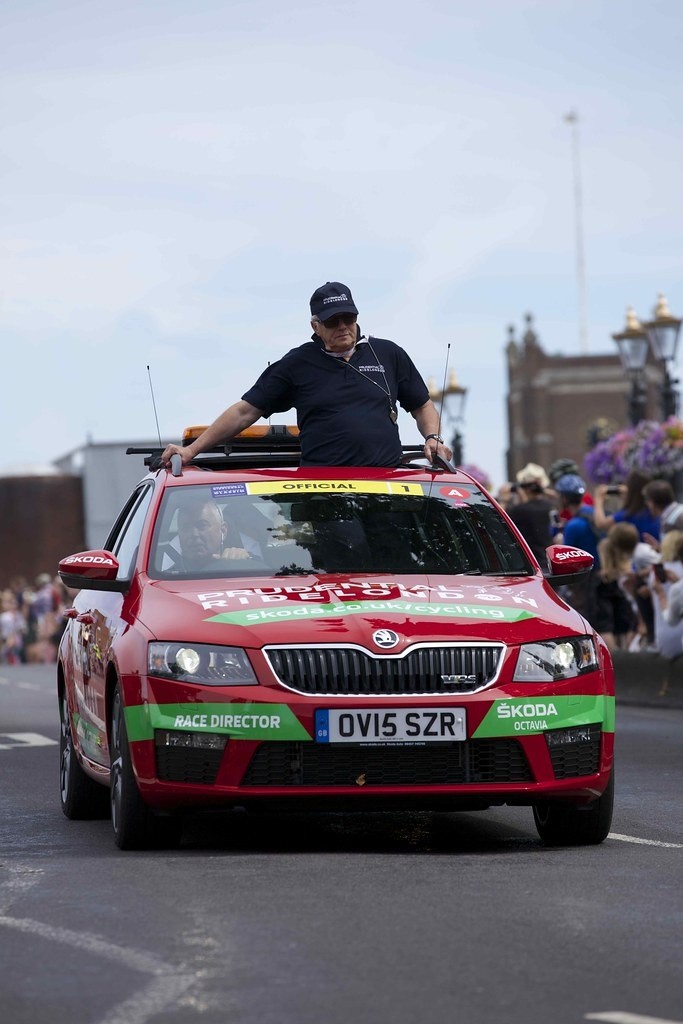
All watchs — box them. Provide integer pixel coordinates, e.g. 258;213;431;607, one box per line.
425;434;444;444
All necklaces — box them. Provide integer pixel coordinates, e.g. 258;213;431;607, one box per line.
323;336;397;425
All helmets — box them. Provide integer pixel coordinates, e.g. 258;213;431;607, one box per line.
549;459;579;484
554;474;587;496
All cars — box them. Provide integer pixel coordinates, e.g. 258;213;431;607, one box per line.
53;426;616;850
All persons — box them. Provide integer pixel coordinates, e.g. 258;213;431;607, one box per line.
495;460;683;659
0;572;83;665
164;500;261;572
162;280;446;571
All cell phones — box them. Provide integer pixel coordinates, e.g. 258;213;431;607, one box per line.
654;564;666;583
606;486;619;494
511;485;517;492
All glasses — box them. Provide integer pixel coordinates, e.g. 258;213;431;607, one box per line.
314;311;358;330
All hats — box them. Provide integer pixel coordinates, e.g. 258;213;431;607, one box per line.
309;282;358;323
516;462;550;490
